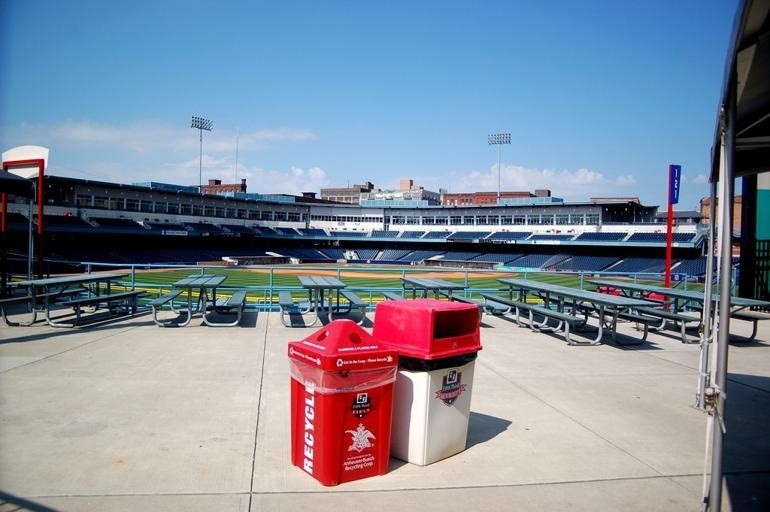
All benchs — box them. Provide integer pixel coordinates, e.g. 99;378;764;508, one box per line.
383;290;407;309
1;291;96;327
54;289;146;324
275;287;298;326
438;288;489;325
340;290;371;328
480;292;659;345
607;295;770;343
145;289;186;327
228;289;249;327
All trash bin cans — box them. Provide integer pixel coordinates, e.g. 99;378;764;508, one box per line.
373;300;483;466
288;319;399;487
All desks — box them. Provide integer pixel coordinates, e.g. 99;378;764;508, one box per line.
173;274;229;326
584;279;770;331
6;274;129;327
401;278;469;301
496;279;663;346
298;276;348;327
